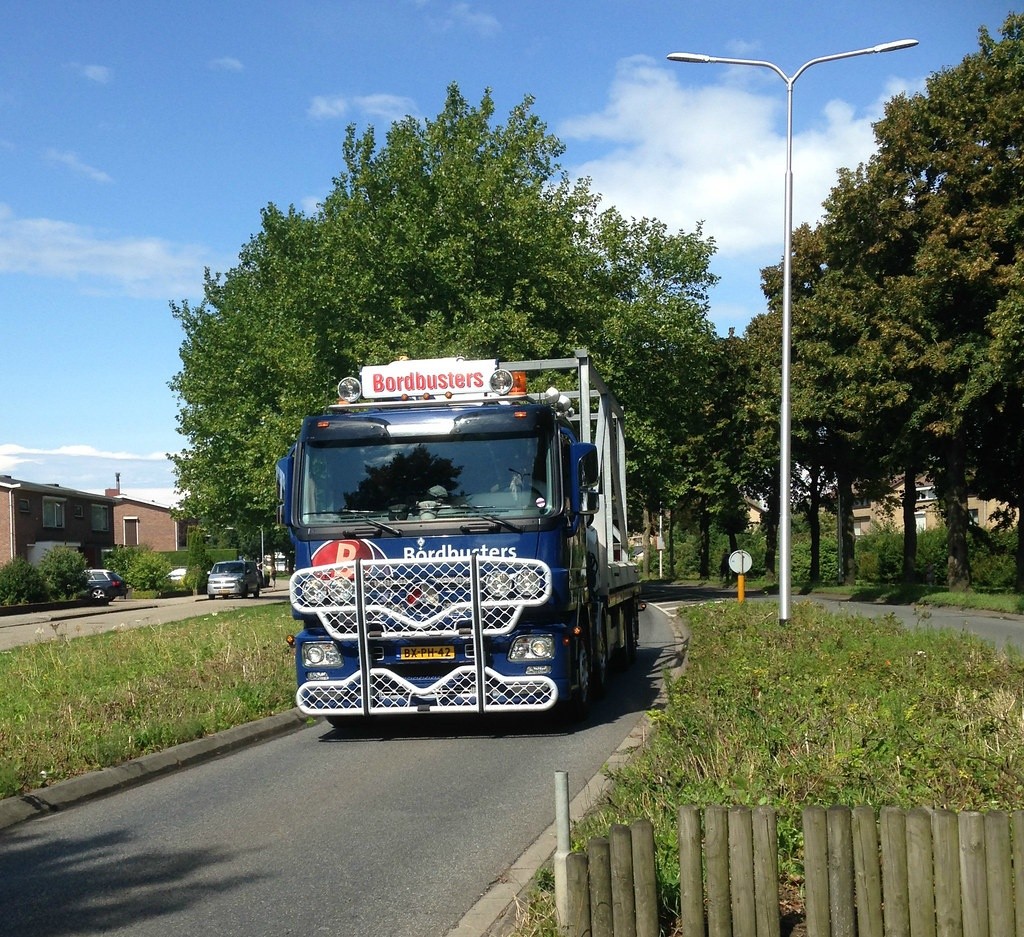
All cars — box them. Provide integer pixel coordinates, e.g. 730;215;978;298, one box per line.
66;569;128;603
162;568;188;582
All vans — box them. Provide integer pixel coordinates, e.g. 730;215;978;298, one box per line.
207;560;263;599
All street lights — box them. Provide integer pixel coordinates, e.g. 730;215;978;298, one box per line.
667;37;921;640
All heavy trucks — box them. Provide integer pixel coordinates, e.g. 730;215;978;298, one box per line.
280;347;643;729
